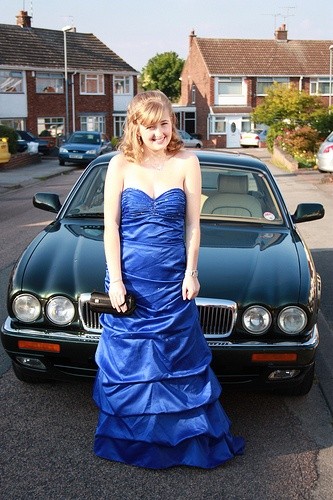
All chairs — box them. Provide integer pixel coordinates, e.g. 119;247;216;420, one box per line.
201;173;263;218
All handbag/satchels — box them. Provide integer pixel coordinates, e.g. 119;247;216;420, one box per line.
87;292;136;319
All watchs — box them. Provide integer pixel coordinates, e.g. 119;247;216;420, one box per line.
184;269;200;278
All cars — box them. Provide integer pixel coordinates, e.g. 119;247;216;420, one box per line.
59;131;113;166
17;131;55;156
240;129;267;148
316;132;333;174
178;130;203;149
0;149;325;396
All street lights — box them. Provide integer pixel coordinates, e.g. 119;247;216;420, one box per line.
329;45;333;115
62;26;74;141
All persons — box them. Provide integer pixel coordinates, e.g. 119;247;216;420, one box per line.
99;90;204;466
